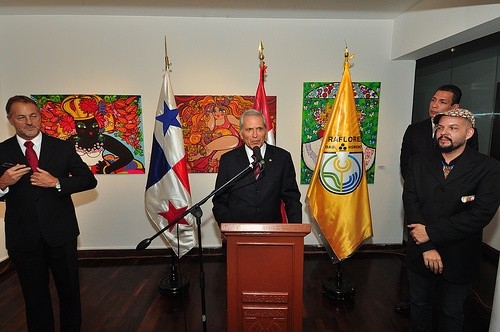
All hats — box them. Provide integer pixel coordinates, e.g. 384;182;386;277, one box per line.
433;108;476;127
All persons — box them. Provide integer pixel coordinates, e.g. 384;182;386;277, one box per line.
0;96;97;332
403;109;500;332
212;108;302;259
393;84;479;314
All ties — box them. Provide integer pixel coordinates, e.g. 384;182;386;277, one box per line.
251;155;260;176
442;161;454;179
24;141;38;172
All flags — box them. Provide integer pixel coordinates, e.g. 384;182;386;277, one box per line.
306;59;373;261
144;71;197;258
254;65;289;224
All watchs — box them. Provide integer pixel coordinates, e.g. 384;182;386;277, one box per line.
55;182;61;191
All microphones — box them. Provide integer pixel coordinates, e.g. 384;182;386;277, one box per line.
253;146;265;164
